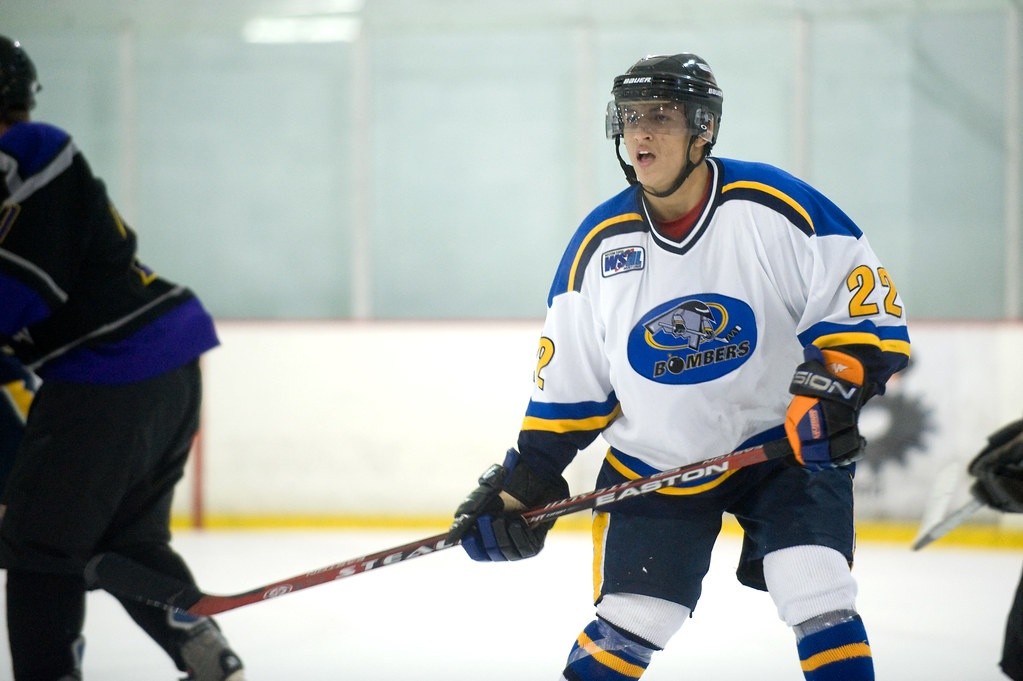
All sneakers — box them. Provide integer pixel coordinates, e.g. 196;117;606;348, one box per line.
179;629;242;681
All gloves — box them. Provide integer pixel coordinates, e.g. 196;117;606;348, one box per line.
783;346;890;473
455;447;570;563
967;417;1022;513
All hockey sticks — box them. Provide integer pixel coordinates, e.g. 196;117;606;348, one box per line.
909;470;987;553
82;437;795;619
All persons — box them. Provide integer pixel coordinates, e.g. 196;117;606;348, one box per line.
454;52;911;681
968;417;1022;681
0;36;247;680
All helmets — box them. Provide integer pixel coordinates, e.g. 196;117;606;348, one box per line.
610;54;724;147
0;33;43;119
